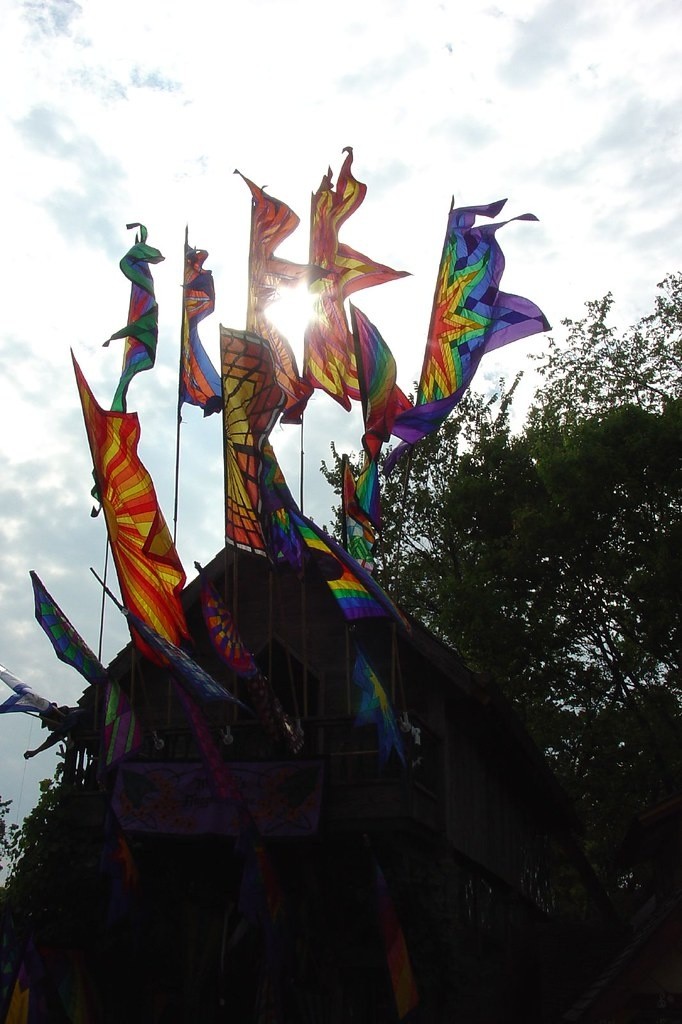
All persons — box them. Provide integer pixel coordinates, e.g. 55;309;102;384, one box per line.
24;702;93;759
